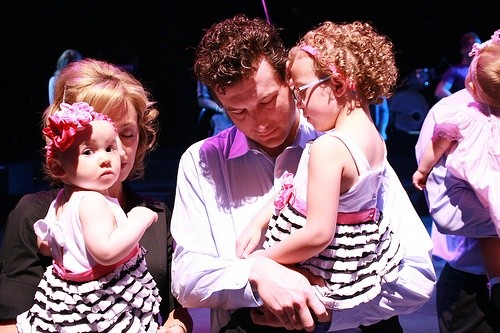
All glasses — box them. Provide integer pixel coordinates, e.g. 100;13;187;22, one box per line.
294;74;332;102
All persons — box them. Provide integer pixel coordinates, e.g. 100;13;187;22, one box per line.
411;38;500;307
218;23;397;333
194;78;232;134
168;16;437;331
16;101;160;333
1;56;195;333
434;31;485;98
370;94;390;141
415;88;500;332
49;50;86;107
